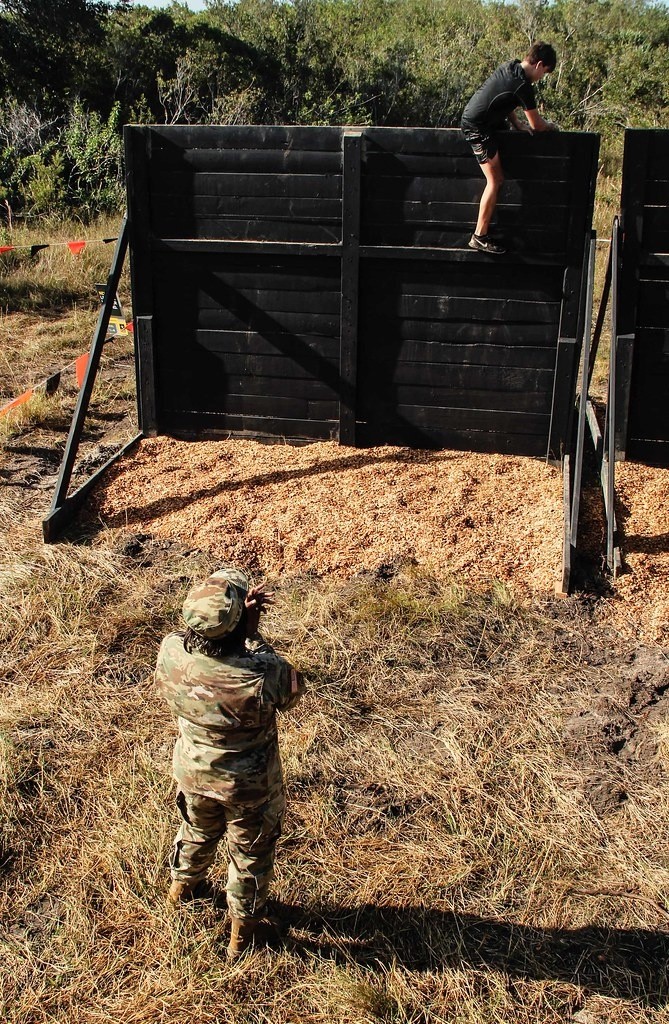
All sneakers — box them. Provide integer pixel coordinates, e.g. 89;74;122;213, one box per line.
469;235;506;254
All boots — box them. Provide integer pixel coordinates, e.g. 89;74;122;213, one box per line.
166;880;196;908
226;910;260;957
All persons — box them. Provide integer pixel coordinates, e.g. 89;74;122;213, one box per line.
156;568;306;956
460;40;558;256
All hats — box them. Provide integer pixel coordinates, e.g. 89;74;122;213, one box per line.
182;569;248;639
